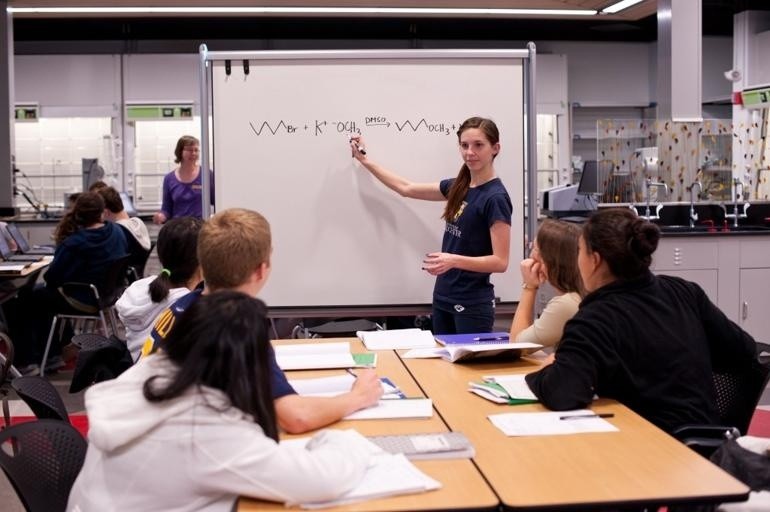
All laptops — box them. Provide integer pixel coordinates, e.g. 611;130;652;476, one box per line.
0;229;44;262
6;222;55;255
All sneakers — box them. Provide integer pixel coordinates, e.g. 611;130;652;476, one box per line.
5;364;39;382
45;355;65;372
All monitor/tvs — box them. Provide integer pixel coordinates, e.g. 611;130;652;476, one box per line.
576;161;615;196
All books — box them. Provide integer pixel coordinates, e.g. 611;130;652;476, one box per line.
363;431;475;460
435;343;542;364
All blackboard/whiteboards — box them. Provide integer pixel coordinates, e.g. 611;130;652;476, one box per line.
197;41;538;322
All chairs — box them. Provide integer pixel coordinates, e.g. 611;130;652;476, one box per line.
34;268;128;382
8;369;75;426
643;335;770;456
1;415;91;512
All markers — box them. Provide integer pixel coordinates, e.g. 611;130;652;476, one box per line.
347;136;369;158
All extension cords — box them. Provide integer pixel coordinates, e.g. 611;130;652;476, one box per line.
10;152;17;198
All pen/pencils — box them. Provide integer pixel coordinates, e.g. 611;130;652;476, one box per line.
559;411;615;421
344;365;358;378
472;335;510;342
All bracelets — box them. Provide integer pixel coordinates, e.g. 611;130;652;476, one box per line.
522;281;538;290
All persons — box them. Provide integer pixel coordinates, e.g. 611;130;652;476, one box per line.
114;218;204;364
91;182;107;197
133;210;382;435
66;286;369;511
350;118;514;336
525;210;760;459
98;187;151;286
152;136;213;226
510;220;586;359
66;191;84;218
4;193;131;377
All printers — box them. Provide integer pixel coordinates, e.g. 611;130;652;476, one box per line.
540;182;579;210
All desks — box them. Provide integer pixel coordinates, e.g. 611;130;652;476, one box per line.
231;335;501;512
392;330;754;512
